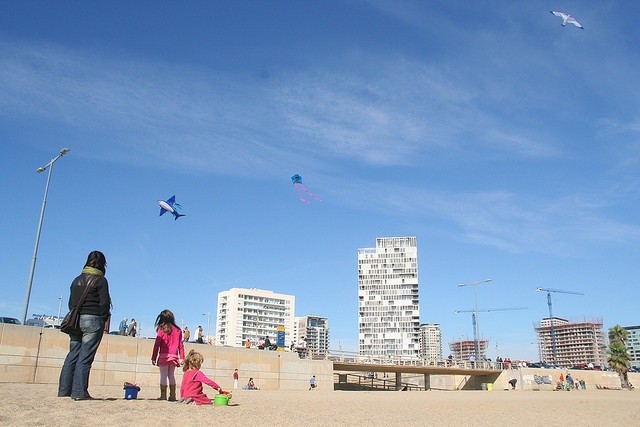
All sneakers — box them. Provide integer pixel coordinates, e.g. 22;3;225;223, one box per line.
184;397;196;405
178;398;185;404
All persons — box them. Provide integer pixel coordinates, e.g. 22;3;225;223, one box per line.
491;356;513;370
151;309;184;400
194;325;202;343
198;328;205;342
232;368;239;389
258;336;273;350
555;370;588;392
243;378;260;390
177;349;224;406
523;362;526;369
445;353;453;367
296;335;310;348
244;338;252;348
183;327;191;342
125;318;137;337
579;360;595;371
58;251;112;401
469;355;475;369
309;374;318;391
118;318;128;337
517;361;522;370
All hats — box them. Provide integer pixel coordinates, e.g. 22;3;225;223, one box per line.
87;251;107;267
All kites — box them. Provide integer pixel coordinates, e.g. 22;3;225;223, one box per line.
155;193;187;224
550;9;585;34
288;172;322;206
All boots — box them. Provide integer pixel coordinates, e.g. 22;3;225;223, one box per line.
169;384;175;400
157;384;166;399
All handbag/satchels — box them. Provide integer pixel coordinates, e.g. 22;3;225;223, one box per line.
60;306;80;333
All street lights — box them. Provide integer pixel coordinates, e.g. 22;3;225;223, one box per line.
20;147;71;326
457;278;494;367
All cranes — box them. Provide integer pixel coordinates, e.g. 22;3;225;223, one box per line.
453;307;530;369
537;286;585;367
